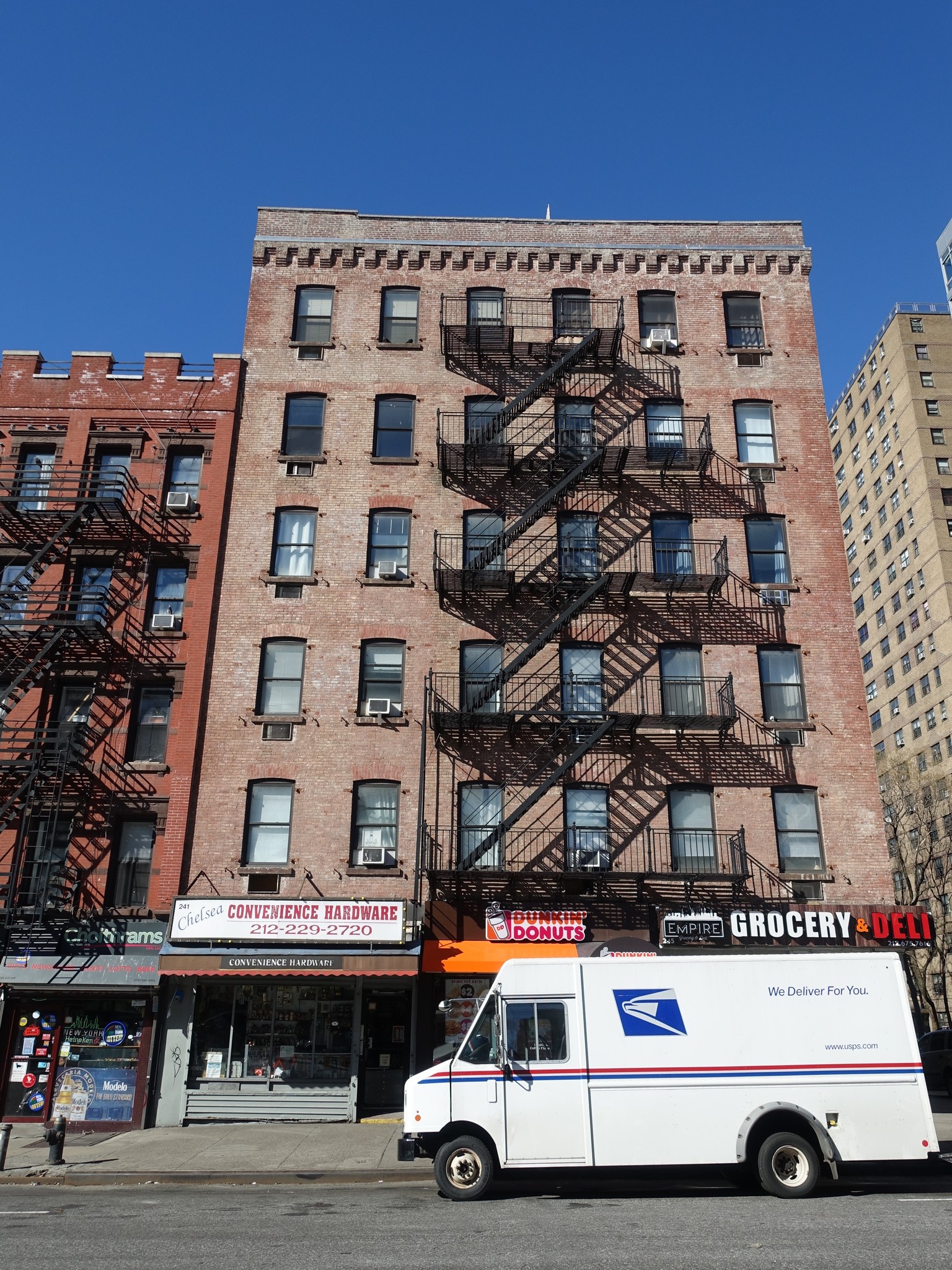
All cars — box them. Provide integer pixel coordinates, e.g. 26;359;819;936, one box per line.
14;1087;46;1116
918;1029;952;1099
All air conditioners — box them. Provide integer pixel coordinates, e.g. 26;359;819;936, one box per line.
368;698;390;715
361;847;385;865
648;329;671;347
378;561;398;578
166;492;191;510
152;614;177;631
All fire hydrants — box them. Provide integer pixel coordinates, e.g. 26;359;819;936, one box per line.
43;1115;66;1165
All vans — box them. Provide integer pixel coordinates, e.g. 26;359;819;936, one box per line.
398;944;940;1199
